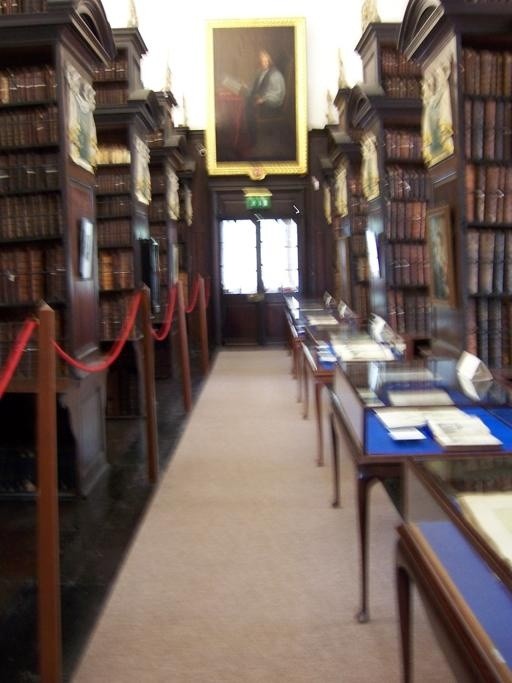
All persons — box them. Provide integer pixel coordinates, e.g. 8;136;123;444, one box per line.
238;46;286;144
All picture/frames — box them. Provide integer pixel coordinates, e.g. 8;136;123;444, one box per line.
205;16;310;181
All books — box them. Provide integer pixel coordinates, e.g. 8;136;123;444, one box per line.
350;175;375;328
146;150;171;309
89;58;134;342
424;412;504;452
381;48;437;337
463;48;511;381
221;77;243;96
359;350;492;431
3;67;68;373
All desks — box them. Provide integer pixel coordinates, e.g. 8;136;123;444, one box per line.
392;490;512;683
326;380;512;624
282;285;357;424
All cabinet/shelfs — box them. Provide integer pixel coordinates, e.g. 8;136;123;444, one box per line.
94;0;197;421
324;0;424;388
0;2;117;498
396;0;511;393
